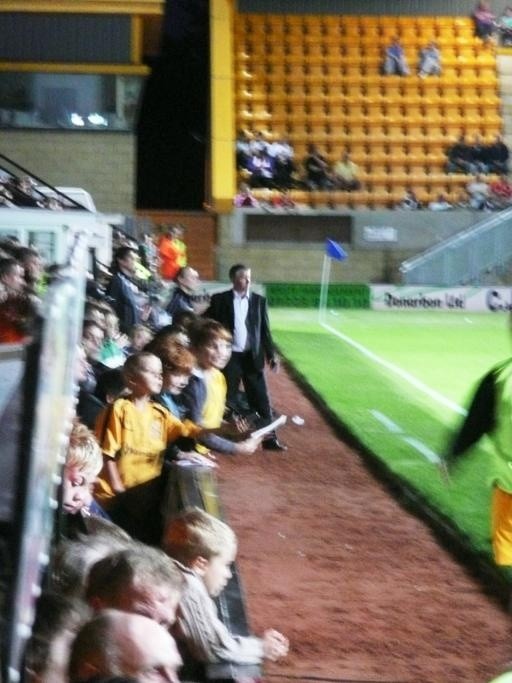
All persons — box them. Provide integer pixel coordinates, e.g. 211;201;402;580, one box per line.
1;234;53;346
417;42;441;78
429;194;453;211
3;175;61;210
403;184;416;209
21;227;289;682
235;140;359;206
466;176;491;207
472;0;501;45
437;309;510;580
499;7;512;46
384;43;409;76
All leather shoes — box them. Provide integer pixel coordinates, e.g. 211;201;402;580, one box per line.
262;437;287;449
